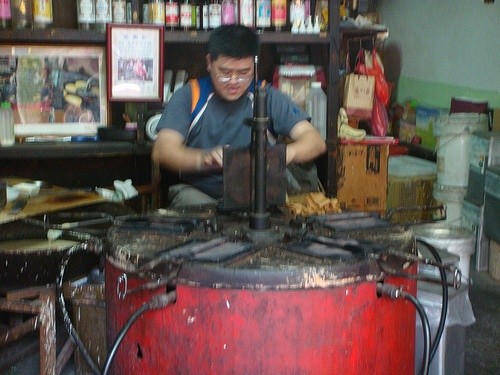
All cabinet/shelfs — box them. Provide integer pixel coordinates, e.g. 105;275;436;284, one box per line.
0;0;341;199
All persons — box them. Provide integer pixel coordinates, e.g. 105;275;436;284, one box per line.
150;25;328;207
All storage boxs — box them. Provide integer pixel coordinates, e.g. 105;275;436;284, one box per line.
337;141;438;223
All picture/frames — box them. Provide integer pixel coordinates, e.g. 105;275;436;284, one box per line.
106;23;163;103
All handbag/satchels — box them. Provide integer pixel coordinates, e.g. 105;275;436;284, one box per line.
342;54;375;119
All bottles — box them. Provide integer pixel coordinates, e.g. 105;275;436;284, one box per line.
76;0;328;34
0;0;54;32
306;81;327;140
0;100;15;146
338;0;360;22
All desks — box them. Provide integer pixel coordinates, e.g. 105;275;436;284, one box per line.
0;177;105;227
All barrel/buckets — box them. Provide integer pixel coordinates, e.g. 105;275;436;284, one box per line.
415;225;475;284
432;111;491;226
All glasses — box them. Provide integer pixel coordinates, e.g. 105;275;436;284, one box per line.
216;72;254;84
